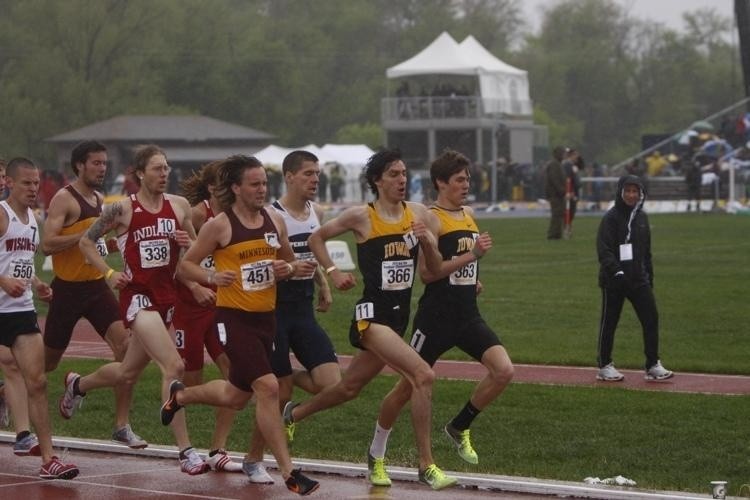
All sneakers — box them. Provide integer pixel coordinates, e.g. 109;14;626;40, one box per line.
179;449;320;495
643;361;673;381
418;465;457;491
596;364;624;382
14;433;41;455
445;418;478;464
0;381;11;429
40;458;79;479
59;372;87;418
110;424;148;449
368;447;392;486
281;401;299;444
160;379;185;425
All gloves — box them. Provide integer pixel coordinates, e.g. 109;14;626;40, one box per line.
615;273;632;295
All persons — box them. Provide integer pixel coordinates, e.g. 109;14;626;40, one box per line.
596;174;673;381
514;114;749;240
1;140;513;496
